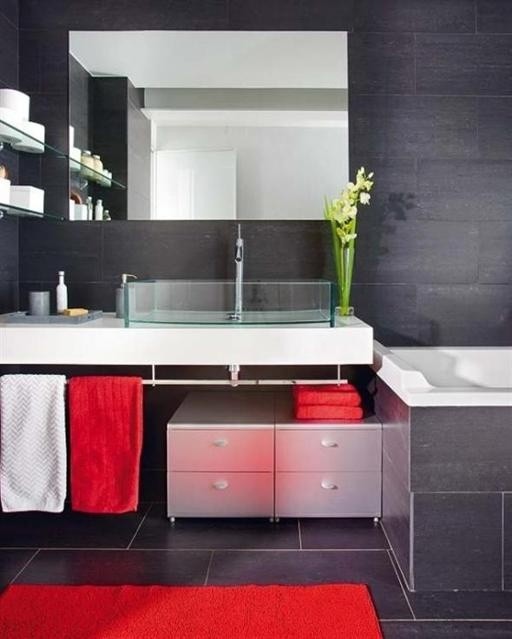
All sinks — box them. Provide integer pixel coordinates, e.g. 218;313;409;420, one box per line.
123;277;336;325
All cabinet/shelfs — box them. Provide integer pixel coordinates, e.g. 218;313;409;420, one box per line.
164;420;387;531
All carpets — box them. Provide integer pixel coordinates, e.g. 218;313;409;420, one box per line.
1;578;388;638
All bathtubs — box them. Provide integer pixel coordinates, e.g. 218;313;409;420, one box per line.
374;340;512;408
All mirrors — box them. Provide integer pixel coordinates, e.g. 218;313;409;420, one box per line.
64;22;353;225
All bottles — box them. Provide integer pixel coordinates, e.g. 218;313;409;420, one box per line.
81;147;114;188
55;271;69;312
83;195;112;221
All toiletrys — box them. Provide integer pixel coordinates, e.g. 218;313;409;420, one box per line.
68;123;115;222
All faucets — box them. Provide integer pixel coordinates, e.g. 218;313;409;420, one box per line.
233;223;243;319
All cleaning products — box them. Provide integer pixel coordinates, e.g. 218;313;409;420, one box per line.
55;270;69;315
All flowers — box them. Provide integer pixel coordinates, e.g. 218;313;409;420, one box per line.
320;166;374;287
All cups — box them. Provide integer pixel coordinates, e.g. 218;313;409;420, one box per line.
28;290;51;316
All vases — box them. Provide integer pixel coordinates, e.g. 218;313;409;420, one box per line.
333;249;358;316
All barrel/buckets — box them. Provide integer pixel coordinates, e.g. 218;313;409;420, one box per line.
28;290;51;316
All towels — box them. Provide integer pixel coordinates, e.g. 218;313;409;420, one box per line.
0;372;146;517
288;382;364;425
